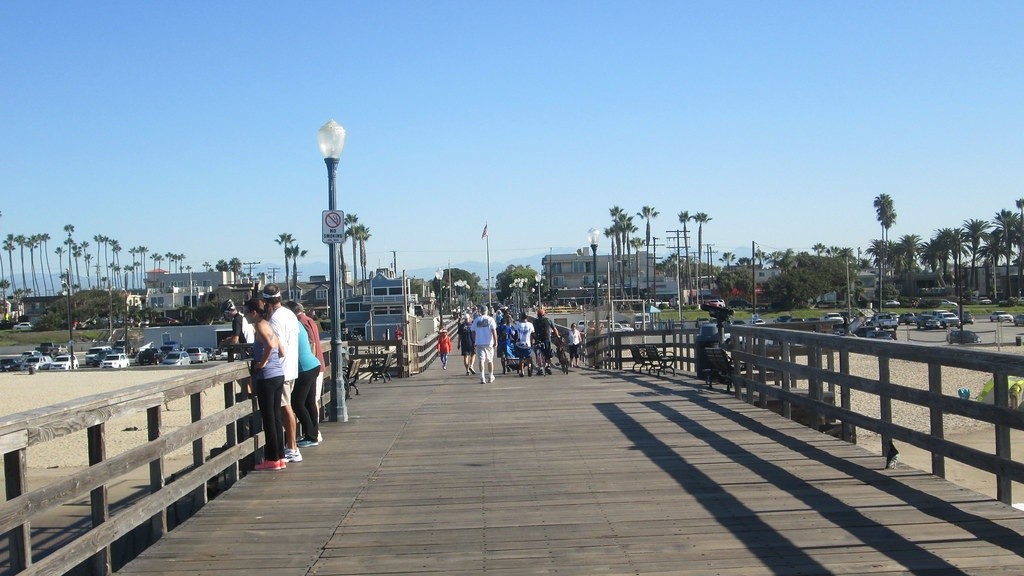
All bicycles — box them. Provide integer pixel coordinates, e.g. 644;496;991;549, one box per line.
532;340;550;376
551;332;572;375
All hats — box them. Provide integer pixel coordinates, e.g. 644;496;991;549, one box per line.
480;306;488;312
464;314;470;319
521;312;528;318
440;330;447;333
538;310;544;315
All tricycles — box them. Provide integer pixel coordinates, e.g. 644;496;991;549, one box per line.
502;342;533;377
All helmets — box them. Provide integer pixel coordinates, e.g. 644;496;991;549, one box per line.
220;298;236;315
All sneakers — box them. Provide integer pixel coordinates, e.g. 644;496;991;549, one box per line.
285;447;302;462
296;439;318;447
255;458;286;470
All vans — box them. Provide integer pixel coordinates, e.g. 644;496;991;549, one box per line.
85;346;111;365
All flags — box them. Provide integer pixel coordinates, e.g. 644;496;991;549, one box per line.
482;225;488;240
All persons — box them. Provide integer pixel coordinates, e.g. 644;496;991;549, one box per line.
457;302;560;383
912;298;919;310
437;330;451;370
220;283;325;470
568;323;583;368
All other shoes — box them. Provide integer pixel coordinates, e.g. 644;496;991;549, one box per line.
507;366;511;372
301;431;322;441
443;366;446;370
469;367;476;374
546;367;552;375
502;372;505;374
528;368;532;377
466;372;470;375
537;370;544;375
575;365;581;368
570;363;573;368
519;373;524;377
481;378;486;384
488;377;495;383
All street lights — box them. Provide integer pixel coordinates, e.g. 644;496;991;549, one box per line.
509;274;543;314
436;268;445;326
586;227;604;369
317;118;349;423
455;279;472;318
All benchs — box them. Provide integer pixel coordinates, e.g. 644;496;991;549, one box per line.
366;353;392;384
629;344;655;374
331;358;362;399
645;345;677;377
701;347;755;391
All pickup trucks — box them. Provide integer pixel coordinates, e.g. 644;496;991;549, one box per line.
139;348;166;365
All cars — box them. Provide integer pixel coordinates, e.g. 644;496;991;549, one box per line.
0;342;79;372
13;322;33;330
577;319;634;333
940;299;957;306
692;308;1023;339
155;317;178;324
884;300;900;306
707;298;751;309
92;339;239;368
946;330;981;344
